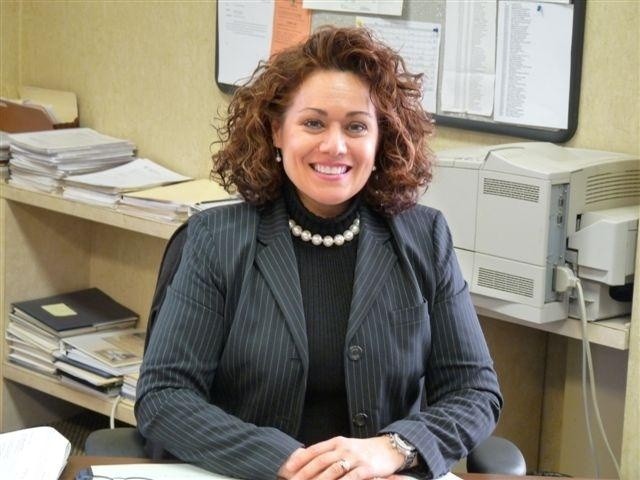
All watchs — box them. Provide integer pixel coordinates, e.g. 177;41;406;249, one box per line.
386;431;418;473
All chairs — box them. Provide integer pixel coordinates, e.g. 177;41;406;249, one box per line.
84;223;527;478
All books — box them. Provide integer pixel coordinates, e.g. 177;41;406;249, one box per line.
0;85;247;225
5;285;147;401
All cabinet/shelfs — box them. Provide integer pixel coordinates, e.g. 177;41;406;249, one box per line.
0;181;189;434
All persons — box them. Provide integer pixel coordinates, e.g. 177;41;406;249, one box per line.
134;27;504;480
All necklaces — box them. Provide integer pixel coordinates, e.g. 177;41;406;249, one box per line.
289;211;361;248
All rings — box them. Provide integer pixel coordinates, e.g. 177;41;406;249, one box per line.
338;456;351;473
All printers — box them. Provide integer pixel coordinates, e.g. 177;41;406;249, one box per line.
409;142;640;324
